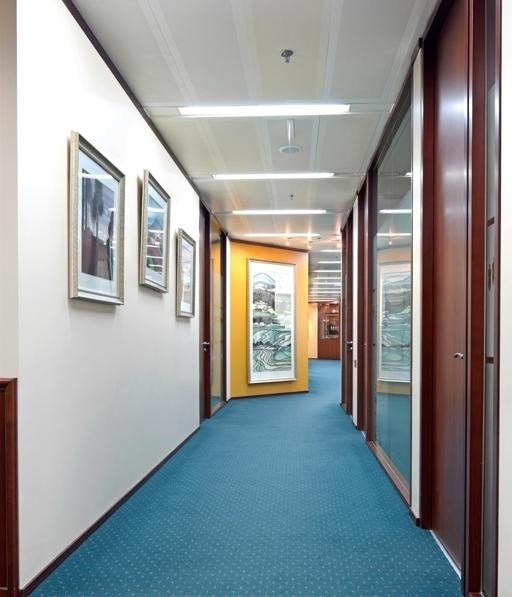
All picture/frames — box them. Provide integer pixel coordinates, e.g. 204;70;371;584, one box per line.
247;257;297;384
376;245;412;396
69;131;197;321
